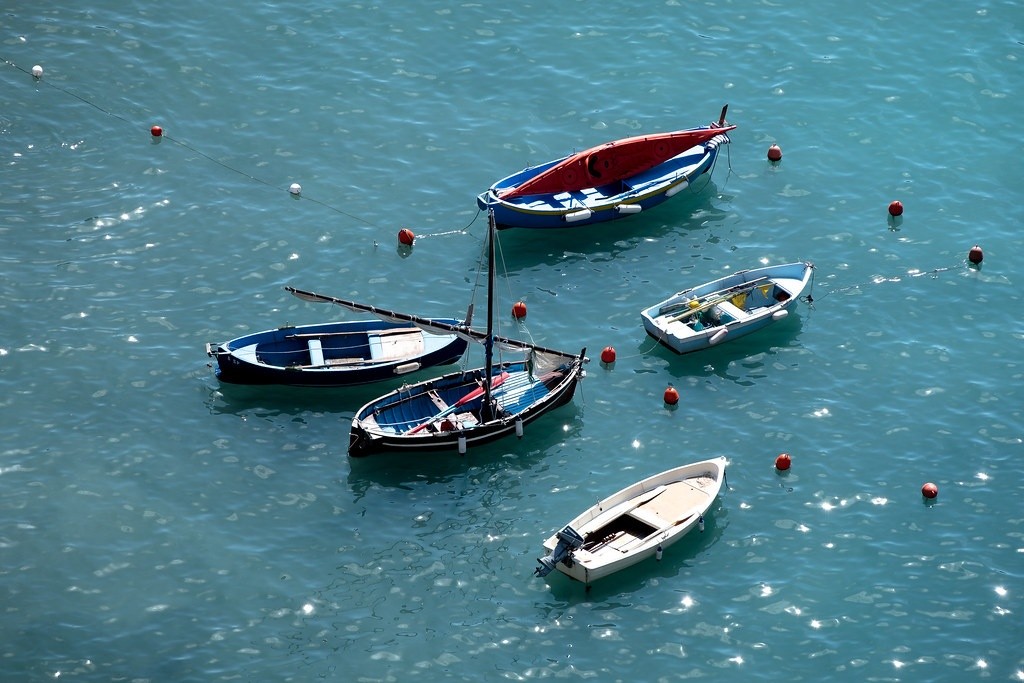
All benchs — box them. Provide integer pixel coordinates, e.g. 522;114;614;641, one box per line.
623;501;673;529
705;294;749;319
308;340;324;365
430;392;463;430
653;314;697;339
568;190;593;206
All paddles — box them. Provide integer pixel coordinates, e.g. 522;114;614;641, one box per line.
300;354;405;366
286;327;426;340
580;484;668;542
660;275;771;317
621;514;695;553
408;372;509;434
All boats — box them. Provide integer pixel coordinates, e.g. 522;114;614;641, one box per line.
477;104;736;232
206;316;469;386
641;261;815;356
532;456;727;584
348;346;588;451
